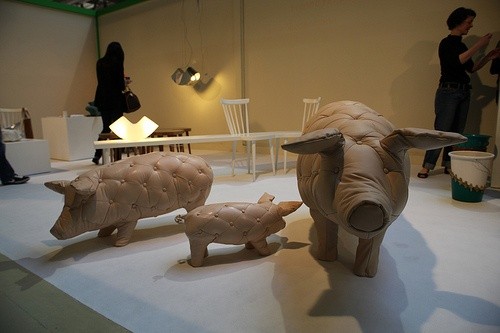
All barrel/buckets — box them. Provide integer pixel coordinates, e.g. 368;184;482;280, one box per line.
452;134;492;151
448;150;495;203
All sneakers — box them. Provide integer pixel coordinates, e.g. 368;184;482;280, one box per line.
2;173;30;185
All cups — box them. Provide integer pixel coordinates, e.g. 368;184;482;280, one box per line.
62;110;68;118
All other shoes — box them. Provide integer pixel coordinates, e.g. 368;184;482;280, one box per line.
92;158;100;165
417;171;428;178
444;166;449;173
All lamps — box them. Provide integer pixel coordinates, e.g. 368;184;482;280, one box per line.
187;67;201;82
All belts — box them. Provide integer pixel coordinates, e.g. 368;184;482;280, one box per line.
441;83;472;90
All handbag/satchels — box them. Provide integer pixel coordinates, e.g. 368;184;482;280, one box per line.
121;86;141;113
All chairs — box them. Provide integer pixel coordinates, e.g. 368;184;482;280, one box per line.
221;96;321;182
0;108;28;131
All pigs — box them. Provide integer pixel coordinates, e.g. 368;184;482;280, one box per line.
43;150;214;247
172;191;303;268
281;100;469;277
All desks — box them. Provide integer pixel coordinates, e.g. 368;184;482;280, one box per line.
93;131;304;177
1;137;51;178
40;117;104;162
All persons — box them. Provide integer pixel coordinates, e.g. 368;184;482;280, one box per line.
416;7;500;178
91;41;131;164
0;129;30;185
